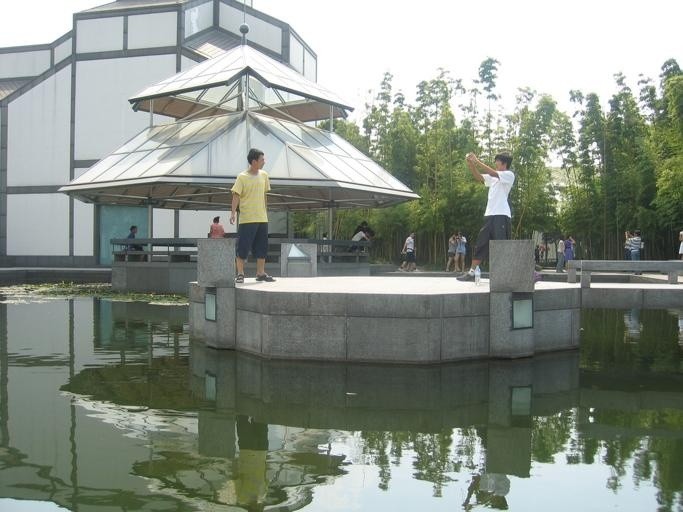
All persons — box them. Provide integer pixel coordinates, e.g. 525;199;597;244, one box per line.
229;148;278;283
445;231;468;273
209;216;225;238
677;231;683;260
622;229;641;275
623;309;644;351
125;226;148;262
461;472;510;510
535;243;545;264
349;221;375;253
556;234;576;273
677;317;683;346
232;415;270;512
457;151;516;283
320;232;328;252
397;232;420;273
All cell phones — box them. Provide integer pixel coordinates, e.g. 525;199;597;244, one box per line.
456;238;459;239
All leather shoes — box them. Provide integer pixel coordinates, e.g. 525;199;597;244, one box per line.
456;274;475;282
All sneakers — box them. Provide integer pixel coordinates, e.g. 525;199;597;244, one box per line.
398;268;420;272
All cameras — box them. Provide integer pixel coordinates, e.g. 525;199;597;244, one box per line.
465;153;472;158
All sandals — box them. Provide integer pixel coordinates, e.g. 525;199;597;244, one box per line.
256;273;274;282
237;274;245;283
